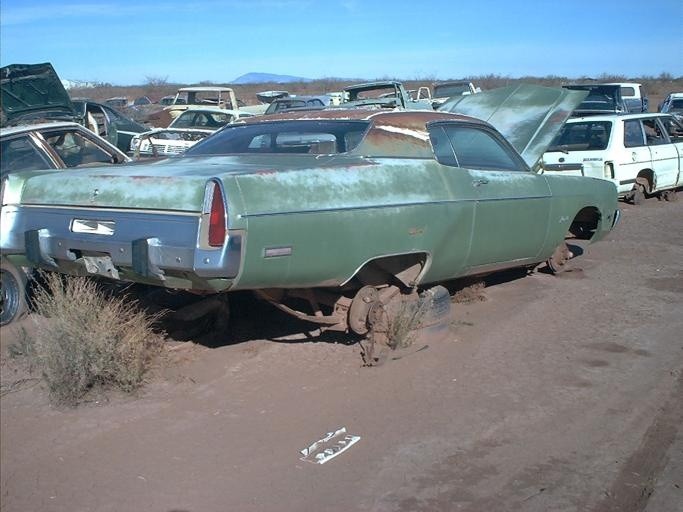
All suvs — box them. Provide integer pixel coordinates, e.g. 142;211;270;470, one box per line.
547;82;649;144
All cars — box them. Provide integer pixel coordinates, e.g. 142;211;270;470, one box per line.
657;93;683;134
0;62;426;330
535;113;683;197
18;106;621;338
432;81;482;111
